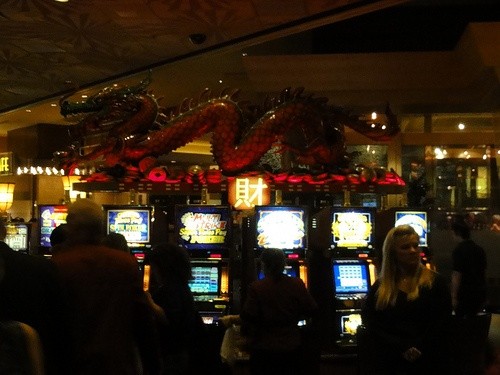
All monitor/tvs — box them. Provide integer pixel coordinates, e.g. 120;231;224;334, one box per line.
4;205;428;300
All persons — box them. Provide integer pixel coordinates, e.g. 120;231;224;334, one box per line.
0;198;209;375
451;220;493;375
221;223;317;375
406;164;435;219
360;226;452;375
